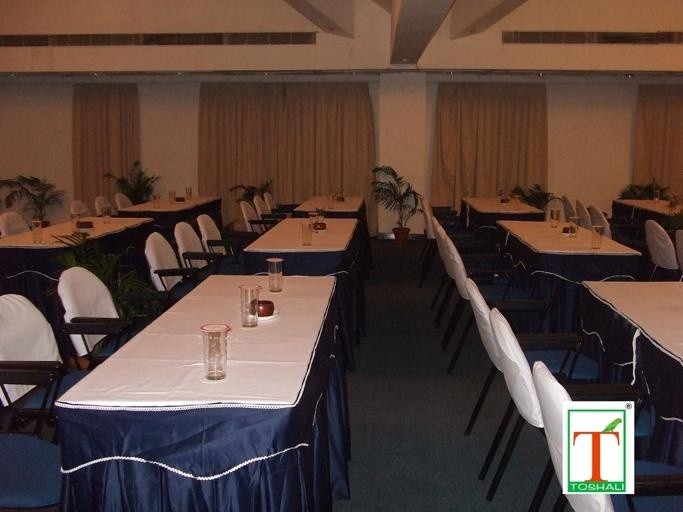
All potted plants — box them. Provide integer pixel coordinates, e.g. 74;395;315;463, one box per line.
0;175;67;231
371;165;424;245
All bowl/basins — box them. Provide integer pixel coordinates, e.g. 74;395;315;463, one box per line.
251;298;273;316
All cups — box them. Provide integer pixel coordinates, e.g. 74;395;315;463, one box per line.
299;210;326;245
31;216;43;244
102;204;112;224
267;258;286;294
237;284;259;327
201;323;230;379
69;207;81;233
549;208;605;252
151;184;193;211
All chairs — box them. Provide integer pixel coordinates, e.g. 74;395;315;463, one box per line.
0;191;682;512
